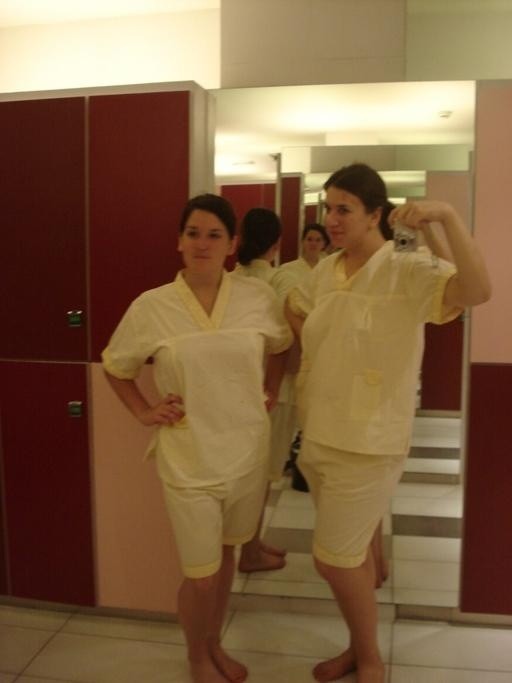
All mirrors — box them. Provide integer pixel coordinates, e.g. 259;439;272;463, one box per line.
197;80;480;609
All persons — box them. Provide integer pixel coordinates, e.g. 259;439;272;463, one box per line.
282;159;492;683
227;205;308;576
368;198;460;590
279;223;327;281
98;192;297;683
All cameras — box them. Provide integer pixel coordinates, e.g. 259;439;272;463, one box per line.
394;218;418;252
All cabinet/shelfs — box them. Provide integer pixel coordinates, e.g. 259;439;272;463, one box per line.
0;78;211;619
